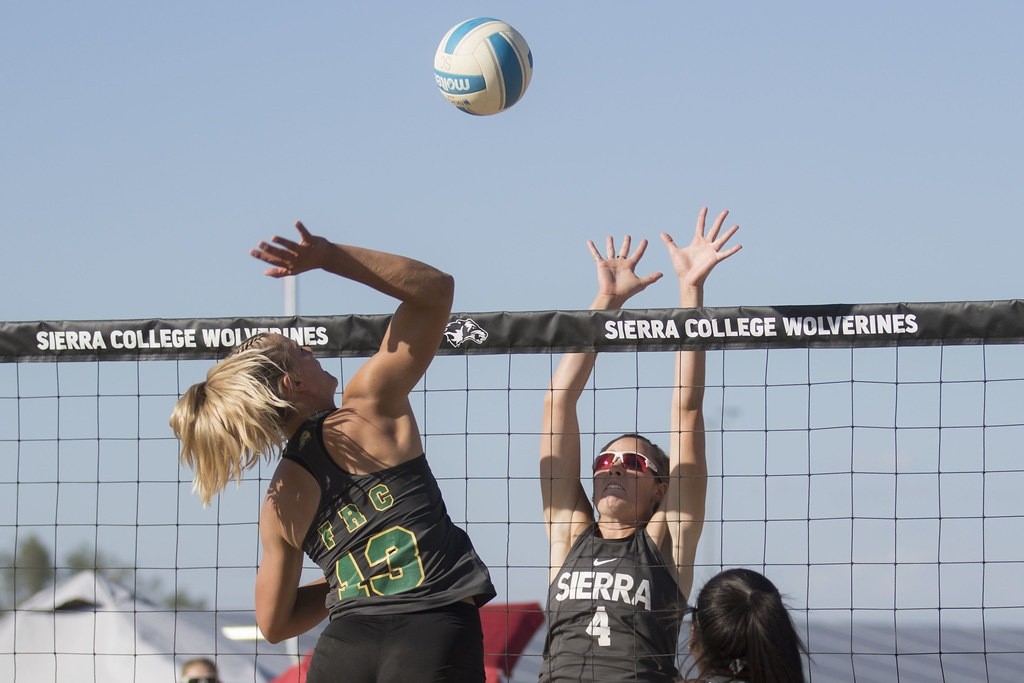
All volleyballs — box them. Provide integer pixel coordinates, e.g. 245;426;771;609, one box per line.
434;15;533;115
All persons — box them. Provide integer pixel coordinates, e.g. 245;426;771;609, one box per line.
177;660;219;683
537;203;743;681
164;219;499;683
673;563;813;683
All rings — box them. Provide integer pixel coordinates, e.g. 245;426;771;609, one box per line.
616;254;627;258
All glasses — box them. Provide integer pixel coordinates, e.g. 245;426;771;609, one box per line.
592;451;664;482
189;677;217;682
